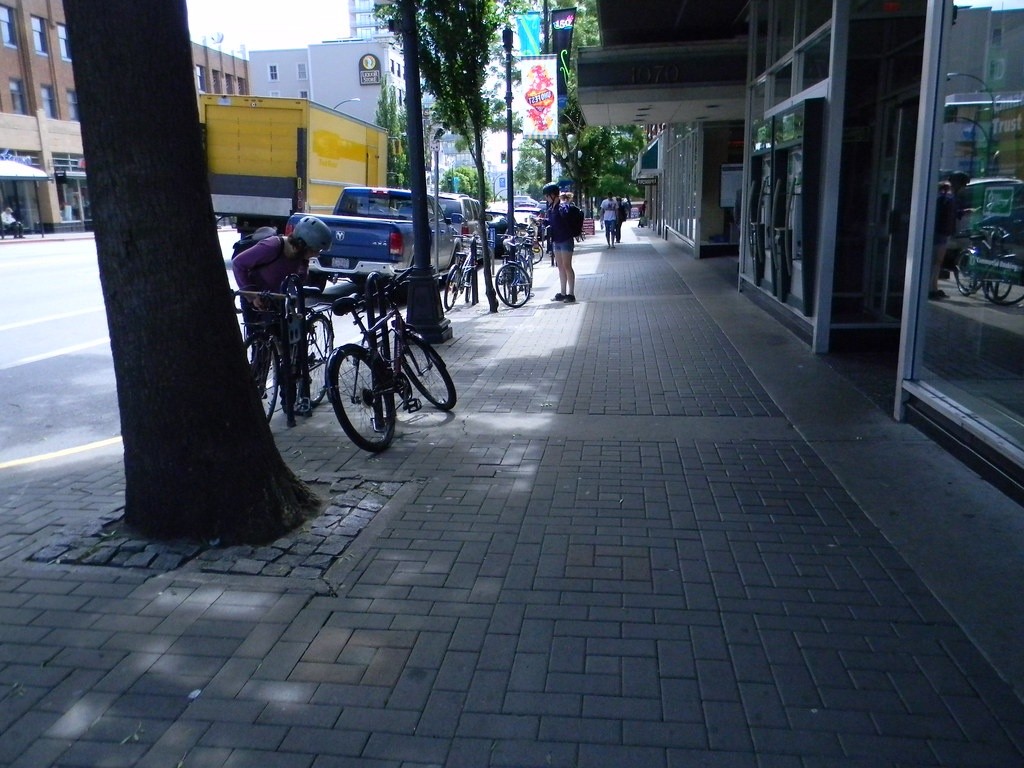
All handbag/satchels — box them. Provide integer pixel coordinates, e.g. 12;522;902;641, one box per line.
640;215;646;224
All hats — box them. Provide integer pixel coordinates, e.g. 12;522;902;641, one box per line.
5;208;13;212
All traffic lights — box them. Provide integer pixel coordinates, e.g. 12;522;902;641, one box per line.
501;153;505;163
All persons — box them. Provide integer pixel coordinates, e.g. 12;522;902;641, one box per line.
1;208;25;239
232;216;333;410
600;192;624;249
534;183;576;302
638;200;648;228
928;171;969;300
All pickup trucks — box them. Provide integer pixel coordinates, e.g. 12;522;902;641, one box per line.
285;186;465;304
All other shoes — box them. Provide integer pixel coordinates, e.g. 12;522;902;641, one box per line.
553;294;566;302
565;294;576;303
607;245;610;249
611;246;615;248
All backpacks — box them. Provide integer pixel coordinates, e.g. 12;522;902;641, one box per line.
233;226;285;270
559;205;584;237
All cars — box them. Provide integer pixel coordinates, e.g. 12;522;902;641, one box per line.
956;178;1024;237
485;197;548;255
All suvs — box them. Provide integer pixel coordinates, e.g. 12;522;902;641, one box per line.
430;193;493;260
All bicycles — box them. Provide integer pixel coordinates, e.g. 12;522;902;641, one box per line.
495;222;543;308
575;229;586;243
444;233;495;310
232;286;334;424
953;226;1024;305
320;267;456;452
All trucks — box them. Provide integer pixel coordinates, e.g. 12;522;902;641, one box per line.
198;93;401;240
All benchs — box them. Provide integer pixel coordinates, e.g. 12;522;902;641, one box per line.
0;226;32;239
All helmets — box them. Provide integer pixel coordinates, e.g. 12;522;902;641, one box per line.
291;216;333;258
542;182;559;196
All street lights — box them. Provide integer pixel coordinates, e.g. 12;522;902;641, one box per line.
947;72;995;178
503;27;514;261
